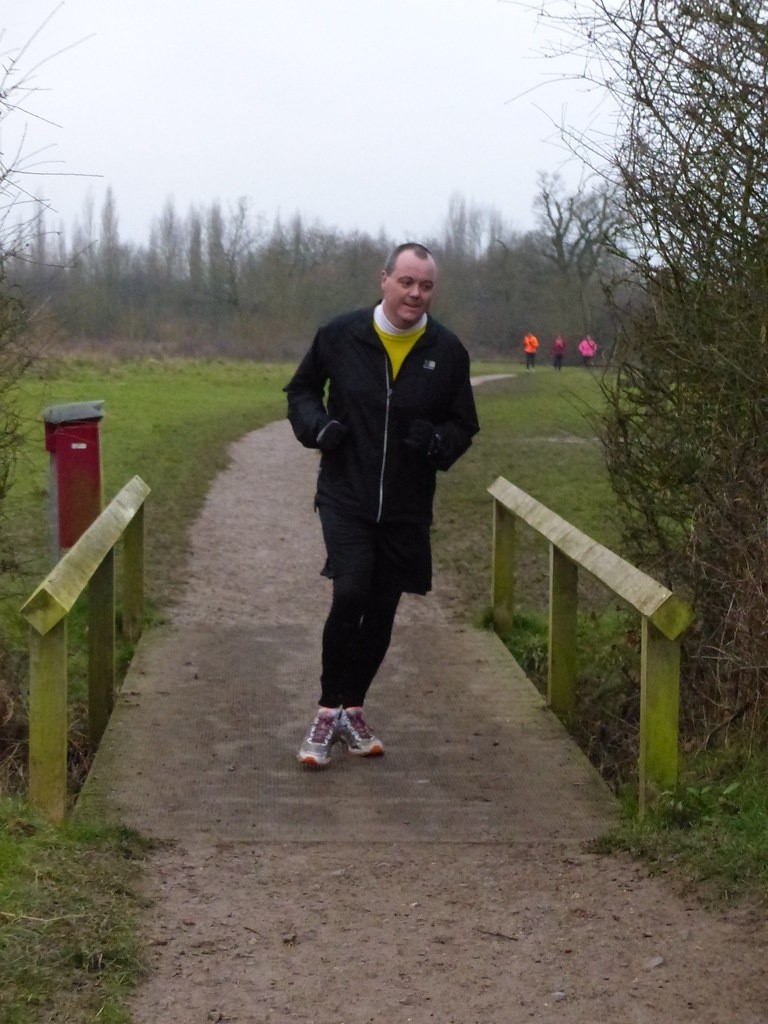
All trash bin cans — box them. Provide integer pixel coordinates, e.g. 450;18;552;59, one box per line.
44;399;105;555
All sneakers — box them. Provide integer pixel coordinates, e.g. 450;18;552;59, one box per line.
339;710;384;756
297;707;344;765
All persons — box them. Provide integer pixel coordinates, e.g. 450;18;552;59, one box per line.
283;241;481;766
553;335;565;370
524;332;539;368
579;335;597;367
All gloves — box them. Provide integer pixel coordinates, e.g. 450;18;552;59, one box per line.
315;421;350;451
403;419;438;459
